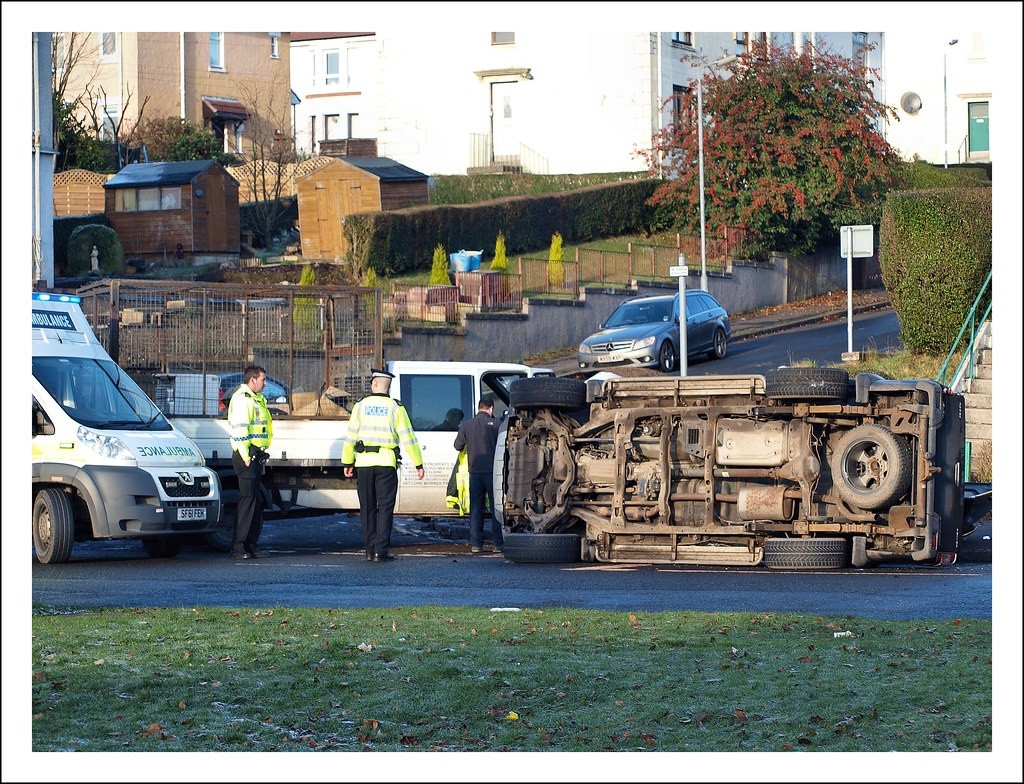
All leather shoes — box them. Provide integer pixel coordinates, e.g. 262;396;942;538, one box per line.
366;553;373;561
372;552;395;561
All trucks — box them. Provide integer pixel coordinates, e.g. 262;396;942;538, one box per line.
76;285;564;557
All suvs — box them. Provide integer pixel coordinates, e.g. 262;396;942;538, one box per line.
575;287;729;373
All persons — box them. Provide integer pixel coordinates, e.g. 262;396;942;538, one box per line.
454;396;501;552
227;365;272;559
340;369;424;562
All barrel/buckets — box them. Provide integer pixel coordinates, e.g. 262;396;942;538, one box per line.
458;249;483;270
450;253;471;272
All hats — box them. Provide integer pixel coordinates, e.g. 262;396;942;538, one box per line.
370;368;396;384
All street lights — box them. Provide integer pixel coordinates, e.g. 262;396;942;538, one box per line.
696;57;741;294
943;38;960;170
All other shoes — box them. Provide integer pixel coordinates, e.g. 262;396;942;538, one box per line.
472;546;483;552
230;552;252;559
246;547;271;557
492;546;502;552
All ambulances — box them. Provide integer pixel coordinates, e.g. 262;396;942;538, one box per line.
30;296;224;572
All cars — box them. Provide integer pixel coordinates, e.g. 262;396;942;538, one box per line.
499;368;992;582
218;371;295;416
